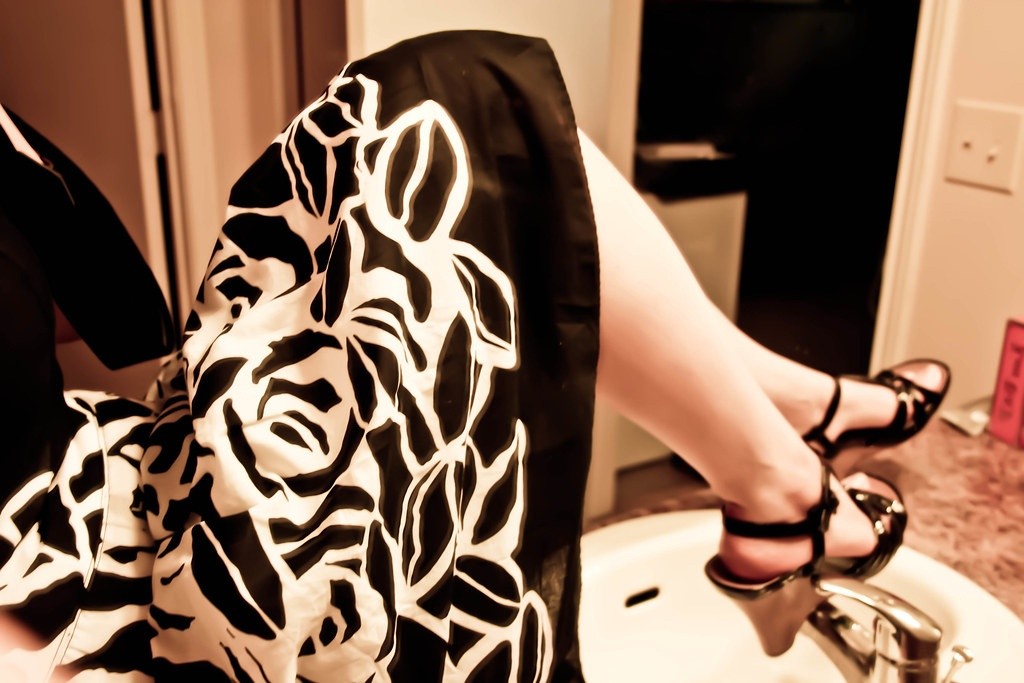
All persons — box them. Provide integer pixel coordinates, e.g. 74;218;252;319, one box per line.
0;29;953;683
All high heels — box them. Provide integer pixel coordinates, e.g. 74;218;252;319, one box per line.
802;358;952;479
705;442;908;657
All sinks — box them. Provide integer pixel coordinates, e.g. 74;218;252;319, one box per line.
577;504;1024;683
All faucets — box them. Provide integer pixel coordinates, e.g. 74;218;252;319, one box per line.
796;575;975;683
